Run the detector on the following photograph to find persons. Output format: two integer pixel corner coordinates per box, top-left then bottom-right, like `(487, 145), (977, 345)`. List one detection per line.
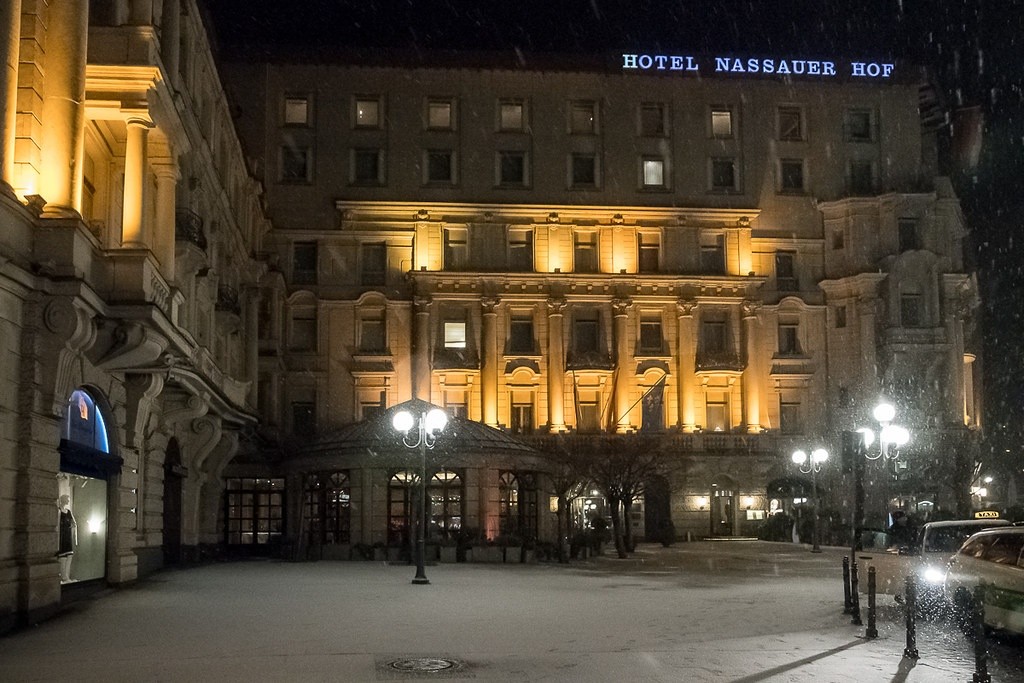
(57, 495), (78, 582)
(757, 504), (934, 551)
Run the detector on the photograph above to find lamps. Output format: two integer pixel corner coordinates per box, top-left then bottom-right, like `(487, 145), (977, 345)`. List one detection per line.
(89, 219), (106, 237)
(24, 195), (47, 217)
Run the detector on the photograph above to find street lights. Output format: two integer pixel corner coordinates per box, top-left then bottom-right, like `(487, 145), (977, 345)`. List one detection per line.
(792, 448), (828, 554)
(392, 408), (450, 587)
(854, 403), (909, 534)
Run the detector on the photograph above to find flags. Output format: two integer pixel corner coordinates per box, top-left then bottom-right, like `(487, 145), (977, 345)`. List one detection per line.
(642, 377), (665, 430)
(575, 387), (583, 421)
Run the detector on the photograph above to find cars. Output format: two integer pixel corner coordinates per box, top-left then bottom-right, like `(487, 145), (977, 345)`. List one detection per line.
(853, 511), (1024, 636)
